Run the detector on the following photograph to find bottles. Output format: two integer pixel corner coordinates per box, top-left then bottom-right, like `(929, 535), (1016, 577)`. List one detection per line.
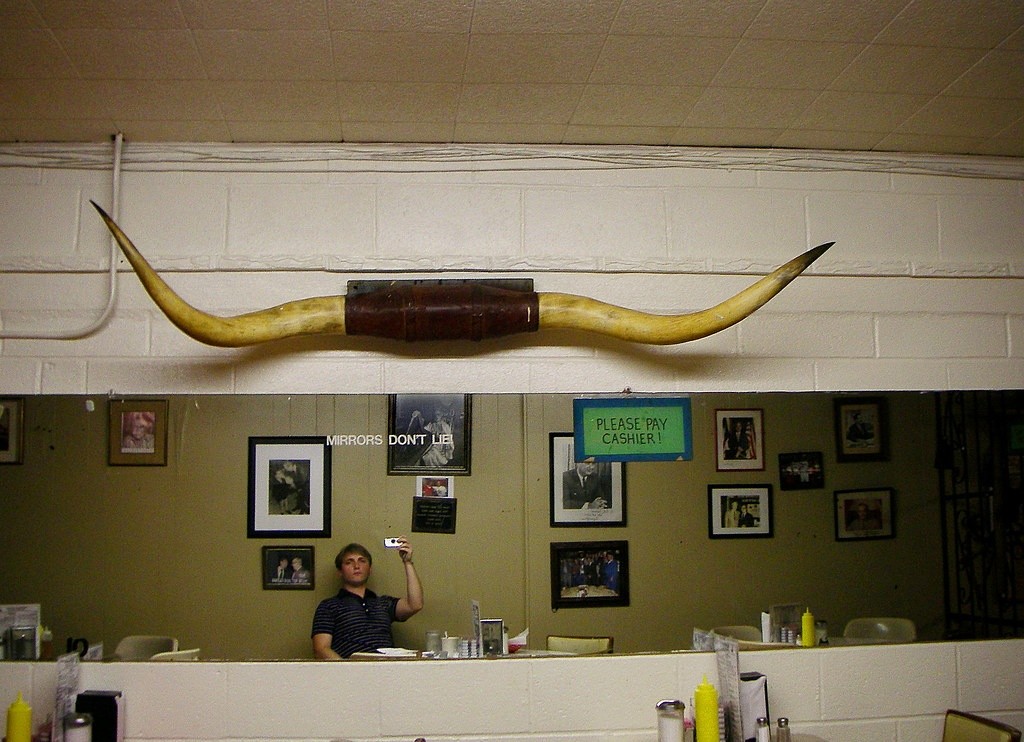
(802, 606), (814, 648)
(654, 698), (686, 742)
(4, 691), (33, 742)
(814, 619), (828, 647)
(63, 713), (92, 742)
(754, 717), (772, 742)
(40, 626), (54, 658)
(776, 718), (792, 742)
(694, 673), (720, 742)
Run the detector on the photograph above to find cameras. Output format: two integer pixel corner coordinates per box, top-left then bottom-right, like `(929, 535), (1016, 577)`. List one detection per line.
(384, 537), (404, 548)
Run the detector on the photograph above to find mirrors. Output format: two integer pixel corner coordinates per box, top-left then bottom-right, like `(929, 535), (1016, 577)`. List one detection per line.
(0, 387), (1024, 663)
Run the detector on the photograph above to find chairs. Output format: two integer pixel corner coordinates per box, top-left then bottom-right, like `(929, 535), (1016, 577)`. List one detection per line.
(111, 634), (202, 663)
(710, 616), (918, 647)
(547, 633), (614, 654)
(942, 709), (1022, 742)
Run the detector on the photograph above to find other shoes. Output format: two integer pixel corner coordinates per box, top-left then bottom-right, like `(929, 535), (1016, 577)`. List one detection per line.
(292, 507), (298, 511)
(299, 511), (304, 514)
(281, 513), (284, 515)
(286, 509), (291, 514)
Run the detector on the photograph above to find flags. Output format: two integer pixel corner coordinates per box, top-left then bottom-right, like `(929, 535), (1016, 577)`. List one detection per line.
(744, 417), (756, 458)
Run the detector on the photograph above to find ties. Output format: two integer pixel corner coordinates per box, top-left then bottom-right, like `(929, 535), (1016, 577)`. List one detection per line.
(280, 569), (283, 580)
(582, 478), (586, 488)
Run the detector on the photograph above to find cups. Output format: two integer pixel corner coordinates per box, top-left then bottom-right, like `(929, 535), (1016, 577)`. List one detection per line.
(441, 636), (458, 654)
(425, 630), (440, 653)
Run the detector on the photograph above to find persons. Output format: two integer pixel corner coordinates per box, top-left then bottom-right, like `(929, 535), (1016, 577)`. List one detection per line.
(121, 414), (154, 449)
(847, 503), (881, 531)
(560, 549), (618, 594)
(562, 460), (609, 509)
(421, 408), (455, 466)
(847, 412), (873, 443)
(268, 558), (311, 583)
(724, 501), (755, 528)
(729, 420), (750, 460)
(272, 462), (310, 515)
(311, 534), (424, 660)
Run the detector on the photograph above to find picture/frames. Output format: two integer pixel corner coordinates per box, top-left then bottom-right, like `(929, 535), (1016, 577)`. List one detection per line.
(416, 475), (454, 498)
(386, 393), (472, 478)
(0, 394), (25, 465)
(108, 399), (168, 467)
(247, 435), (332, 538)
(831, 396), (892, 463)
(550, 540), (630, 609)
(832, 488), (896, 542)
(549, 431), (628, 528)
(261, 545), (315, 591)
(707, 483), (774, 539)
(779, 451), (824, 492)
(715, 407), (765, 472)
